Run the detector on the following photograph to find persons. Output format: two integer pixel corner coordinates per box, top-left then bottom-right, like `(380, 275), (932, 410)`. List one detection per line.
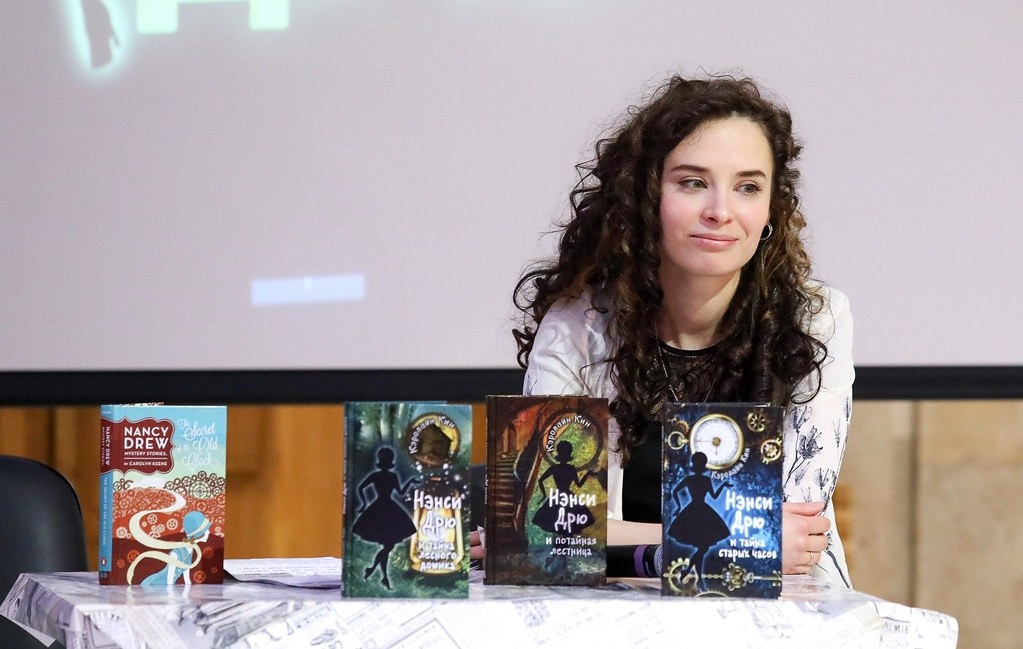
(513, 71), (855, 593)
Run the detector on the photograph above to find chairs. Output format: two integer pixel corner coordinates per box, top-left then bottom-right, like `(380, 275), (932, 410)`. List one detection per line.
(0, 454), (88, 649)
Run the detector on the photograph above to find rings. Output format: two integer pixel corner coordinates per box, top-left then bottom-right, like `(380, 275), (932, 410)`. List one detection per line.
(806, 551), (814, 565)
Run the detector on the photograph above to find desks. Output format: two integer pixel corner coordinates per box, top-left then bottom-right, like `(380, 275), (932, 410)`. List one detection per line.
(1, 565), (960, 649)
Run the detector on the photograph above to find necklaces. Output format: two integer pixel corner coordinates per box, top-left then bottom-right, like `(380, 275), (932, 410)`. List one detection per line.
(653, 315), (736, 404)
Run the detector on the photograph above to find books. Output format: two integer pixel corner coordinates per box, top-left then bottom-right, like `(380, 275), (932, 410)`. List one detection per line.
(98, 406), (229, 589)
(661, 400), (785, 599)
(484, 394), (613, 587)
(338, 399), (474, 600)
